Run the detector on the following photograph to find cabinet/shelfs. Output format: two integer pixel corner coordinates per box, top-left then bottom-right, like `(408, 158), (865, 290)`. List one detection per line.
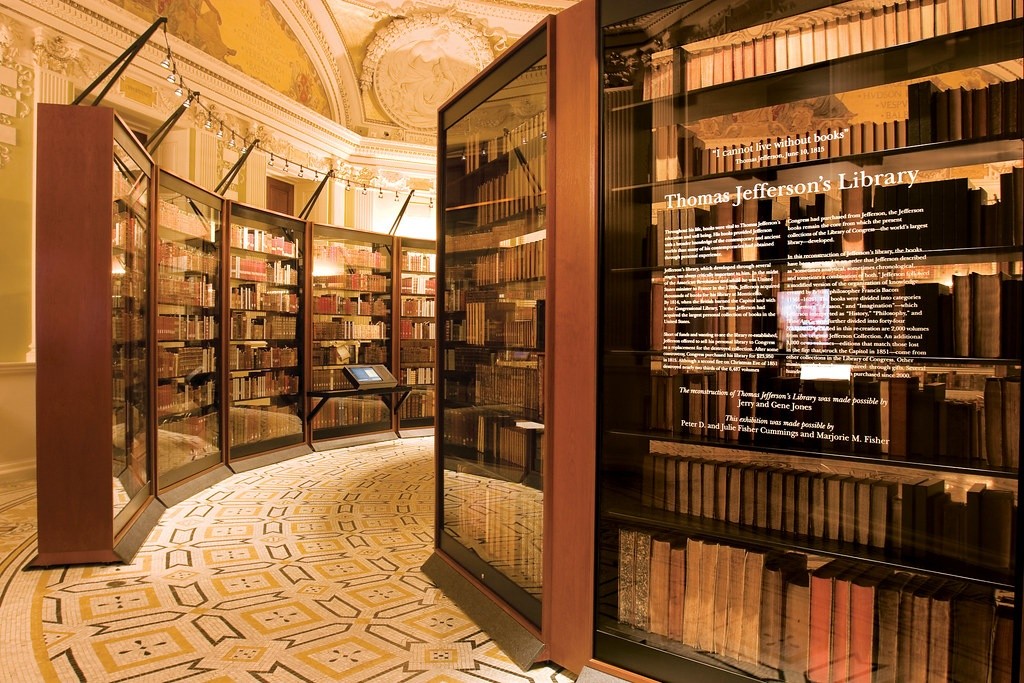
(417, 0), (1024, 683)
(308, 220), (400, 452)
(22, 102), (166, 571)
(226, 199), (313, 474)
(155, 164), (235, 507)
(394, 235), (437, 439)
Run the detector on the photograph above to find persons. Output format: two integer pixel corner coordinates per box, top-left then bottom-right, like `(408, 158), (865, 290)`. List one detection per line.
(783, 290), (826, 352)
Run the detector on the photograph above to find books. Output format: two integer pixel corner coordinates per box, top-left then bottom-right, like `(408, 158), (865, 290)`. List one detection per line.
(113, 0), (1024, 683)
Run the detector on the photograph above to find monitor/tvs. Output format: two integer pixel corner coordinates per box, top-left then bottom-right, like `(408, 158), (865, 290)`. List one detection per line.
(349, 367), (383, 382)
